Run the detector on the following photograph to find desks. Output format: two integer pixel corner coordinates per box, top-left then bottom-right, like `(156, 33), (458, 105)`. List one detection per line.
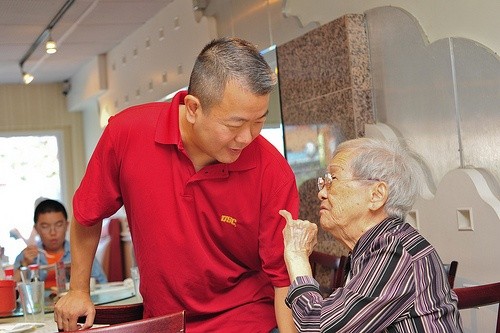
(0, 277), (147, 333)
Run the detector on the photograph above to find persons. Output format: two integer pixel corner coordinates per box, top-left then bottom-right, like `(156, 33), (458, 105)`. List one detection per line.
(54, 37), (300, 333)
(279, 137), (463, 333)
(12, 199), (107, 291)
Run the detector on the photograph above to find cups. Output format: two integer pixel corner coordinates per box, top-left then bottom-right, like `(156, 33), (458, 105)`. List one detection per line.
(17, 280), (45, 325)
(130, 266), (143, 303)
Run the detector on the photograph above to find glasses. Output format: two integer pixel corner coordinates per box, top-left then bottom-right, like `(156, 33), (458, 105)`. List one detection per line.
(318, 173), (379, 191)
(34, 222), (66, 234)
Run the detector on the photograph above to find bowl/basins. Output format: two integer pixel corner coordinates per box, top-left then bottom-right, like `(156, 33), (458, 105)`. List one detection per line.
(0, 279), (17, 317)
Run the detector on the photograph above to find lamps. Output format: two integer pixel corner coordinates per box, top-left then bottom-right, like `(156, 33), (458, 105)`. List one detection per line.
(20, 65), (34, 85)
(46, 28), (57, 54)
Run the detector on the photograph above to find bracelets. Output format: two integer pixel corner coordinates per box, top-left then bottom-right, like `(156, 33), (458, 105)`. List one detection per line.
(16, 260), (23, 271)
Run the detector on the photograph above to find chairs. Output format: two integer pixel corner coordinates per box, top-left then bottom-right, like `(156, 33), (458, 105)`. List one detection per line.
(95, 219), (125, 283)
(71, 309), (187, 333)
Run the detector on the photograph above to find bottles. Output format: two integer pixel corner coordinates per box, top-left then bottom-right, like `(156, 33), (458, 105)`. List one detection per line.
(28, 264), (43, 282)
